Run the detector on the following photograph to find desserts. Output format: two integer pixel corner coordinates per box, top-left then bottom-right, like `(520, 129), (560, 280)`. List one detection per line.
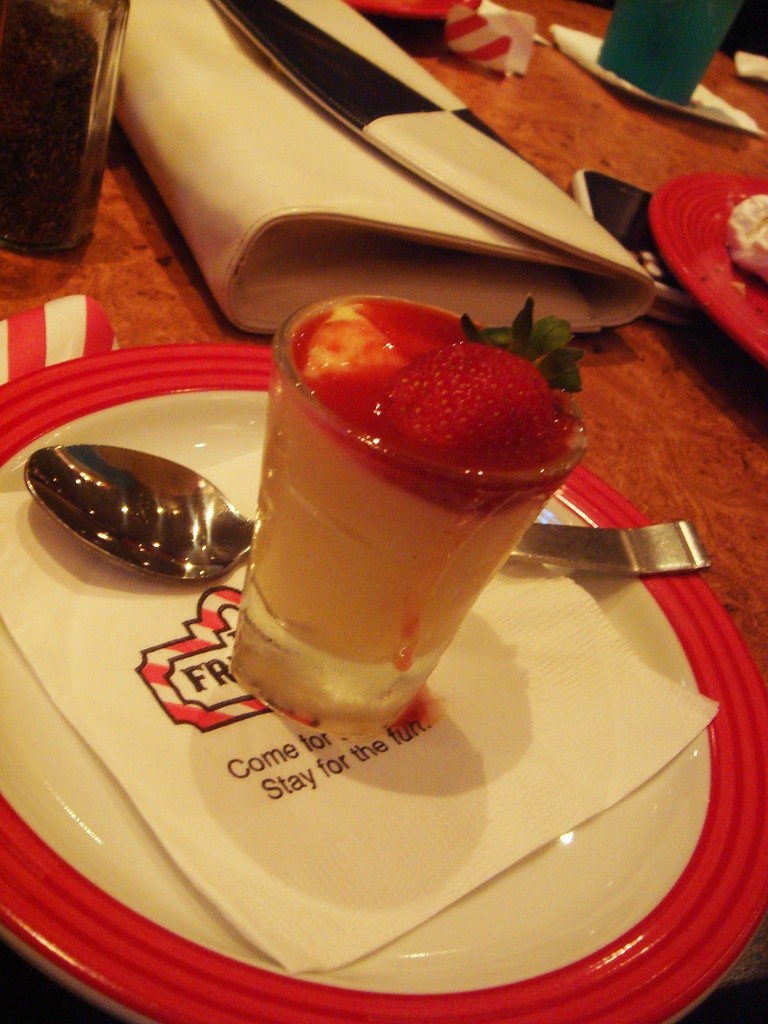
(725, 193), (768, 286)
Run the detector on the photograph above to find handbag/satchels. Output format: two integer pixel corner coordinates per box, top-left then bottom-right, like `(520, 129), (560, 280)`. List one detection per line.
(112, 1), (656, 334)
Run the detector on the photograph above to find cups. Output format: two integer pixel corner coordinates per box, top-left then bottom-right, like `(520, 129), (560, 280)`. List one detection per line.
(230, 296), (588, 737)
(0, 0), (132, 253)
(597, 0), (743, 106)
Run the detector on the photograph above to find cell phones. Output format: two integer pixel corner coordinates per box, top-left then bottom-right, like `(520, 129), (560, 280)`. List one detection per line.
(568, 167), (706, 329)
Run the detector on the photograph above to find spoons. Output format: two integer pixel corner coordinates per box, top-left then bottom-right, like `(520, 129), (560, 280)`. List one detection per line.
(24, 443), (712, 584)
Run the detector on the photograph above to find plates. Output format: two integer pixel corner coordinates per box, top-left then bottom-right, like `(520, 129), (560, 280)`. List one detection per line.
(648, 172), (768, 369)
(0, 343), (768, 1024)
(342, 0), (482, 33)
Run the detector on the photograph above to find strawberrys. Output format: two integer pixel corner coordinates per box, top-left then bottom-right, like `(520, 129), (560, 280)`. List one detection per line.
(387, 304), (586, 461)
